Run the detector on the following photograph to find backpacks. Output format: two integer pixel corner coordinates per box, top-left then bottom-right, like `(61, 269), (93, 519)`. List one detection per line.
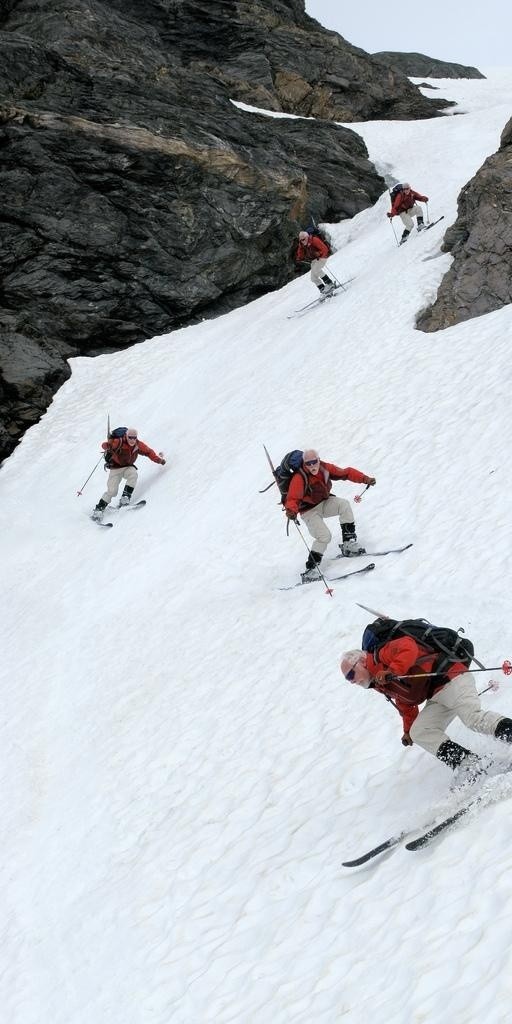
(390, 182), (413, 215)
(361, 616), (474, 701)
(275, 449), (329, 510)
(104, 426), (138, 470)
(306, 226), (331, 259)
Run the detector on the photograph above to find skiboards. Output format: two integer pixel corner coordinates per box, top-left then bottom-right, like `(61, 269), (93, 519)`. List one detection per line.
(390, 216), (442, 251)
(286, 276), (356, 319)
(340, 767), (512, 867)
(275, 545), (412, 591)
(89, 500), (146, 528)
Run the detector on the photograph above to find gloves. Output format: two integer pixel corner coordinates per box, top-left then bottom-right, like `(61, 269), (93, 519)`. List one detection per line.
(366, 478), (376, 486)
(401, 733), (413, 746)
(286, 509), (297, 520)
(375, 670), (393, 686)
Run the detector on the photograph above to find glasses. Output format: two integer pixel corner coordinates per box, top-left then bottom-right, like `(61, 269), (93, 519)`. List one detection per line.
(344, 657), (360, 681)
(127, 435), (139, 440)
(302, 459), (319, 466)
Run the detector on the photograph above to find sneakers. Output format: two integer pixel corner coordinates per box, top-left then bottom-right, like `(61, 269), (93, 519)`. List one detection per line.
(302, 568), (330, 582)
(342, 538), (367, 553)
(450, 751), (483, 792)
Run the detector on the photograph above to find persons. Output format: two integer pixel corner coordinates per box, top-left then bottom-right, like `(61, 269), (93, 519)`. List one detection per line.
(339, 617), (511, 787)
(89, 423), (166, 522)
(287, 448), (375, 578)
(385, 183), (428, 243)
(296, 230), (334, 302)
